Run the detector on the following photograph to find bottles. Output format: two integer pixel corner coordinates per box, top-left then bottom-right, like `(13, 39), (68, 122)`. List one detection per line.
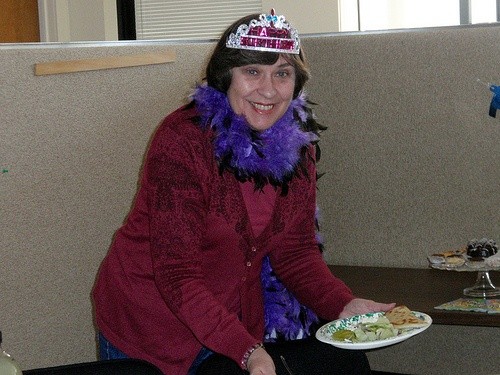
(0, 331), (23, 375)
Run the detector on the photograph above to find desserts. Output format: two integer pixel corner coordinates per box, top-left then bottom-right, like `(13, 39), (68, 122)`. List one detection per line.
(427, 238), (500, 268)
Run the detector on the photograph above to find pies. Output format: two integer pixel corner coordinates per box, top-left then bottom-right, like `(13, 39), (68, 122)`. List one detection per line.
(381, 304), (427, 325)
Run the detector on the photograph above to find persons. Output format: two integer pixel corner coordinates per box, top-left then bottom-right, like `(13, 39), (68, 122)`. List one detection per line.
(90, 8), (396, 375)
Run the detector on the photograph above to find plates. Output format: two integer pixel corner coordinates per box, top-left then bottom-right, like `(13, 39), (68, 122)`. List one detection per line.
(316, 310), (432, 348)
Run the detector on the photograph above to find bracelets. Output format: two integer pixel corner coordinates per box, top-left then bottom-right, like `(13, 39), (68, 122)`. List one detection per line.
(241, 342), (265, 371)
